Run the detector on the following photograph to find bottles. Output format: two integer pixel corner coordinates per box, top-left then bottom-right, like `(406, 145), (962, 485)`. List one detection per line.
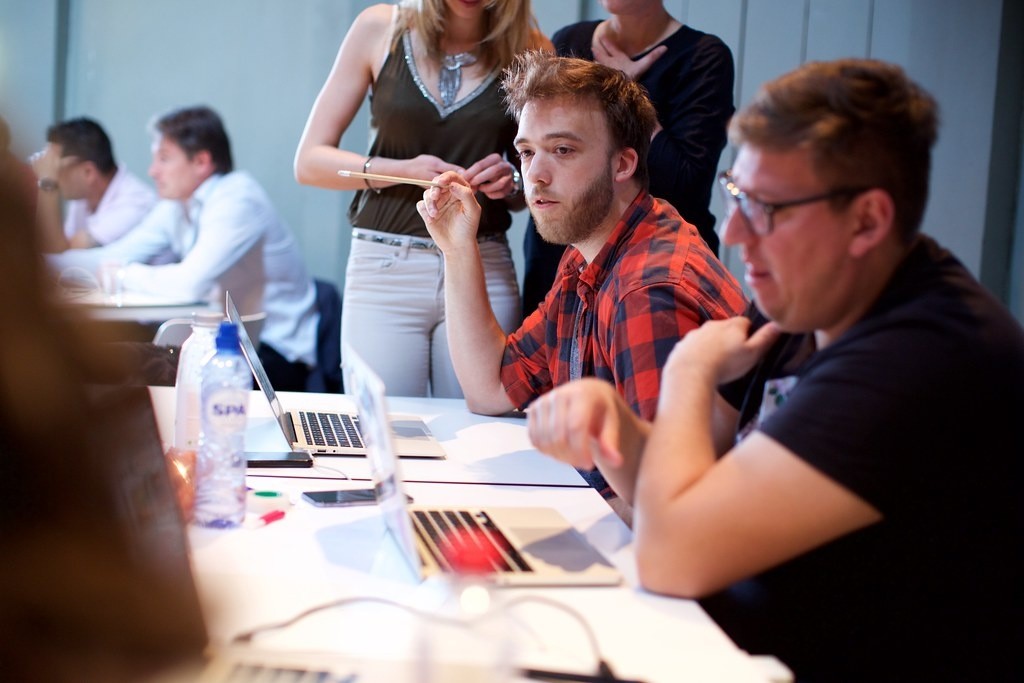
(175, 313), (225, 452)
(192, 322), (252, 527)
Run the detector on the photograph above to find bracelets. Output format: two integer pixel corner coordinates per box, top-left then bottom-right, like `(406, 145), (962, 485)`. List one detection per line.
(512, 164), (523, 191)
(37, 178), (59, 192)
(363, 156), (381, 195)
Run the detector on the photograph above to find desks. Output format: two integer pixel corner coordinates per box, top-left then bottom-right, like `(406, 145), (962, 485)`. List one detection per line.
(149, 389), (782, 682)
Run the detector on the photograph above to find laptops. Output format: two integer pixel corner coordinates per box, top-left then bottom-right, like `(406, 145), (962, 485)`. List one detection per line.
(345, 346), (625, 585)
(223, 288), (447, 463)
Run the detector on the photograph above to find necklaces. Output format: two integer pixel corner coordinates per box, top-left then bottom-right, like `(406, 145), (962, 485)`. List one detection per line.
(439, 51), (477, 106)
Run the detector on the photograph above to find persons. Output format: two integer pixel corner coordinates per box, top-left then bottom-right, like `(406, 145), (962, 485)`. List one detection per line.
(527, 54), (1024, 683)
(521, 0), (737, 328)
(417, 46), (751, 532)
(0, 147), (214, 682)
(28, 119), (183, 266)
(292, 0), (558, 400)
(44, 107), (319, 393)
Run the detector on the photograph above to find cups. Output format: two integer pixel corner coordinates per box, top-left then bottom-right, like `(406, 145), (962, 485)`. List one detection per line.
(100, 265), (126, 295)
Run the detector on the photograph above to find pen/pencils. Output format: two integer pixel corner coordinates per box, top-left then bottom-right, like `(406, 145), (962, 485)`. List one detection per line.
(252, 498), (296, 531)
(250, 486), (288, 498)
(337, 170), (450, 187)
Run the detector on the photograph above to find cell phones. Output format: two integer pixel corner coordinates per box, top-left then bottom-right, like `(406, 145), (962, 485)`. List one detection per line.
(300, 487), (411, 508)
(226, 452), (313, 469)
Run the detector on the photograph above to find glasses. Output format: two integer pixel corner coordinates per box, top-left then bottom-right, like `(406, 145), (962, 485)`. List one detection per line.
(717, 169), (868, 236)
